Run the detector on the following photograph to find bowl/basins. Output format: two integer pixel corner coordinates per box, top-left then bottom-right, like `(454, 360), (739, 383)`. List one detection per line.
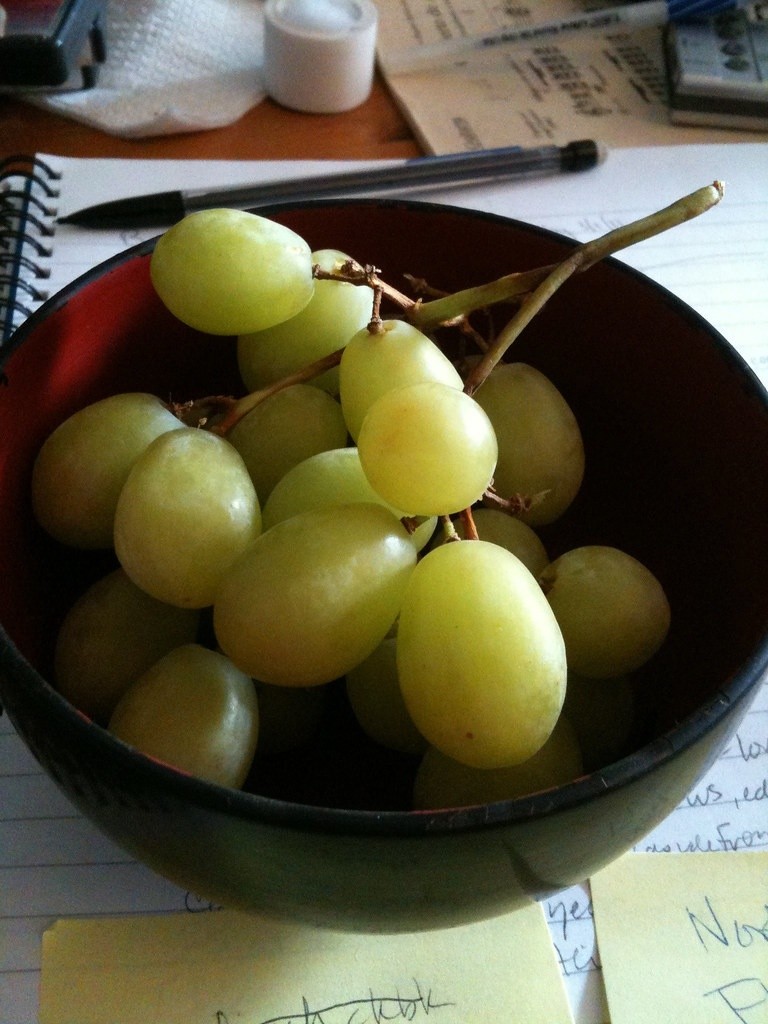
(0, 197), (766, 935)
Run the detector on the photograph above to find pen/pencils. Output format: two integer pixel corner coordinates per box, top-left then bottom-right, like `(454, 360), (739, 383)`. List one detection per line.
(390, 2), (668, 78)
(53, 137), (597, 233)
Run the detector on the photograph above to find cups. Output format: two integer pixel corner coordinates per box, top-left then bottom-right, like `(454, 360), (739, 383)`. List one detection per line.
(262, 0), (379, 115)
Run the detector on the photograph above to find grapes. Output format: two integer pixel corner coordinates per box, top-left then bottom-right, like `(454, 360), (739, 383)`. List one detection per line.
(35, 207), (671, 816)
